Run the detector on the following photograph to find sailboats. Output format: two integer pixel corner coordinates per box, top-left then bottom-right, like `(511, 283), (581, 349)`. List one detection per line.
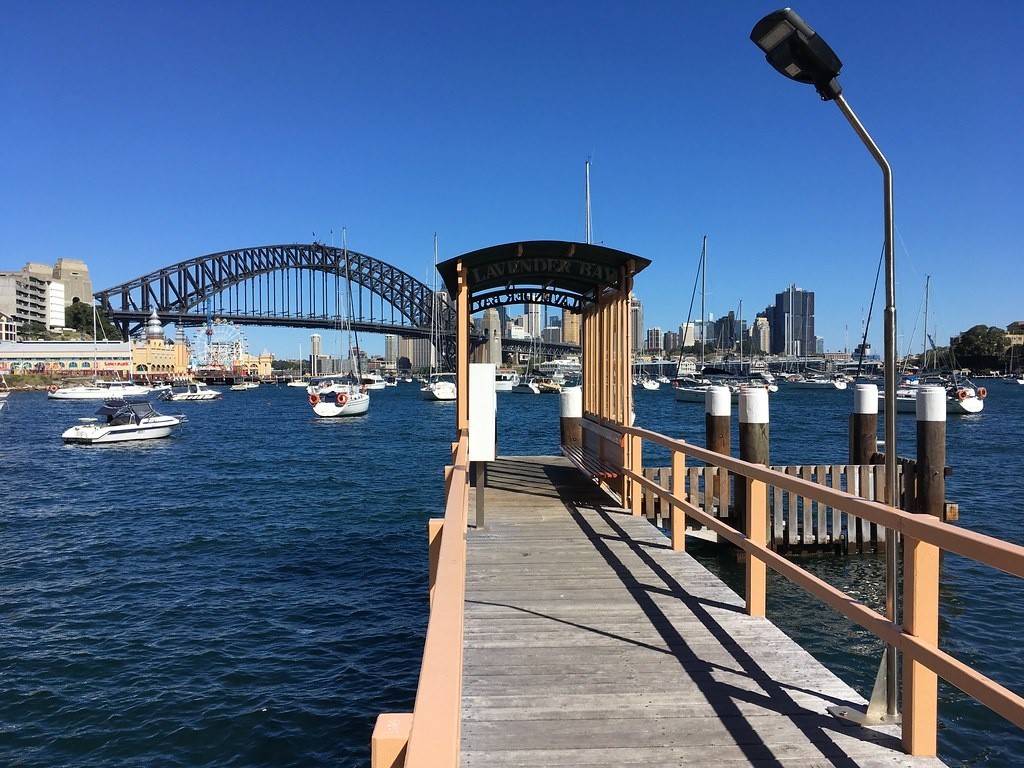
(308, 227), (370, 417)
(512, 158), (964, 401)
(1000, 330), (1024, 384)
(243, 354), (259, 389)
(418, 233), (457, 402)
(877, 275), (987, 415)
(47, 295), (172, 399)
(317, 294), (359, 394)
(287, 340), (310, 387)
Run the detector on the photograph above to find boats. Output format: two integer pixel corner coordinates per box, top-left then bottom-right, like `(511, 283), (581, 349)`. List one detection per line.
(320, 373), (386, 391)
(230, 385), (246, 391)
(495, 367), (520, 391)
(851, 317), (881, 361)
(385, 375), (424, 387)
(156, 381), (222, 401)
(61, 395), (189, 444)
(539, 360), (582, 387)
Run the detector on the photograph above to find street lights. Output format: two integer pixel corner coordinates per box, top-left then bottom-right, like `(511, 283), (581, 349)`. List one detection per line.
(750, 5), (901, 715)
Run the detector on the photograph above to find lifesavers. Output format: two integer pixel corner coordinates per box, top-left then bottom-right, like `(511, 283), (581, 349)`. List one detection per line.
(979, 388), (987, 398)
(309, 394), (319, 404)
(959, 390), (967, 400)
(51, 386), (58, 392)
(337, 394), (348, 404)
